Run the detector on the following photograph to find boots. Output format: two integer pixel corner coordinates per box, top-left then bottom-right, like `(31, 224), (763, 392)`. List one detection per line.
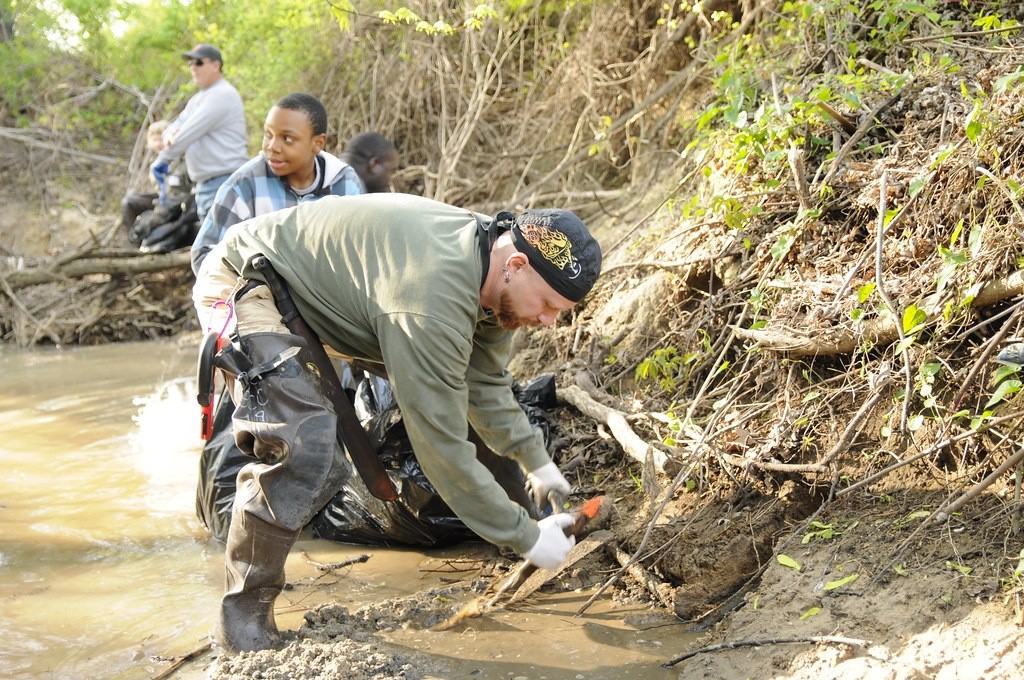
(207, 504), (302, 655)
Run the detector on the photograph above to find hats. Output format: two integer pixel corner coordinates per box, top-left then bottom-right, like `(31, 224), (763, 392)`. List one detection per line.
(489, 208), (601, 304)
(181, 44), (223, 63)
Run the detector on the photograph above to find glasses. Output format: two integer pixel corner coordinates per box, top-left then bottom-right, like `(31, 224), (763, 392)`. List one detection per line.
(187, 58), (212, 66)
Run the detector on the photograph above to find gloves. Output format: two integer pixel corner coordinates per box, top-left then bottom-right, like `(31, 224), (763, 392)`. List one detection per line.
(149, 158), (169, 186)
(524, 463), (571, 517)
(520, 512), (575, 571)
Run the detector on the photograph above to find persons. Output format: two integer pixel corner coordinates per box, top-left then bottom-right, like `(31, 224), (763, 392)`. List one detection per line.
(192, 93), (363, 277)
(341, 132), (399, 192)
(120, 121), (201, 253)
(148, 43), (250, 226)
(191, 192), (603, 657)
